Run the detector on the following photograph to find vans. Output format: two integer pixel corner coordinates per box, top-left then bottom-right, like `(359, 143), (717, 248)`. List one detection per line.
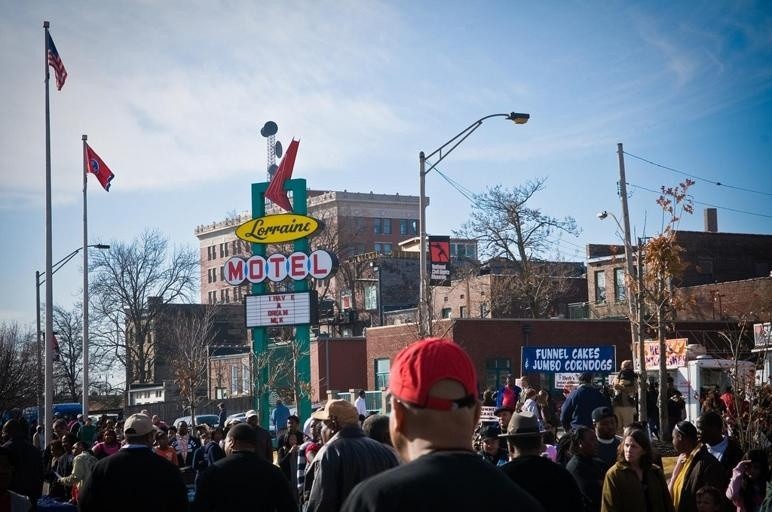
(85, 413), (118, 427)
(173, 414), (223, 430)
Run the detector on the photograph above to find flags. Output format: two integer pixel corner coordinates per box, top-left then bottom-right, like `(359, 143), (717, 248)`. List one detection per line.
(45, 30), (68, 89)
(84, 141), (115, 192)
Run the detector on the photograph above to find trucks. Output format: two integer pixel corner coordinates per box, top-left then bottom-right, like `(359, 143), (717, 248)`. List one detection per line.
(614, 360), (757, 437)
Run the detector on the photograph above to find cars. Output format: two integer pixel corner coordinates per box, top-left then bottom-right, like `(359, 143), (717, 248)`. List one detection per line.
(221, 403), (320, 432)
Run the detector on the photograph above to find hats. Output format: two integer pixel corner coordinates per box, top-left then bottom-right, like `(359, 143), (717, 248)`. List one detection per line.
(591, 407), (616, 423)
(217, 403), (224, 408)
(382, 337), (479, 411)
(311, 398), (360, 424)
(494, 406), (513, 416)
(479, 425), (501, 442)
(244, 410), (258, 419)
(497, 412), (552, 437)
(123, 413), (157, 438)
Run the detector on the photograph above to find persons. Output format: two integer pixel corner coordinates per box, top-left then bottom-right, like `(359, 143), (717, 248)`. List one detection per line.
(473, 360), (772, 512)
(341, 338), (548, 509)
(1, 388), (400, 511)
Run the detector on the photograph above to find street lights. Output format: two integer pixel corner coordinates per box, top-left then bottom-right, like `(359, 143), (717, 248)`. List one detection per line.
(34, 241), (110, 439)
(417, 112), (530, 342)
(596, 209), (627, 235)
